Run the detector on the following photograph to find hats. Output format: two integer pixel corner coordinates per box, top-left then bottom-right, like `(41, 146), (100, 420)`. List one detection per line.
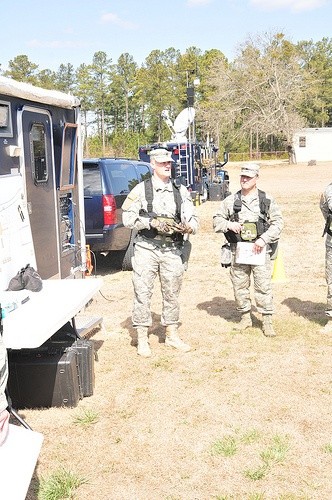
(239, 164), (259, 178)
(150, 149), (175, 163)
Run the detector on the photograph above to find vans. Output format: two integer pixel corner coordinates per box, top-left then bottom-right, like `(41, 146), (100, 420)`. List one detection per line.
(83, 157), (154, 270)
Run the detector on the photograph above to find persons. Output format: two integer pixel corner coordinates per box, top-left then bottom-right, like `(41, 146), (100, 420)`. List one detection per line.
(212, 162), (285, 337)
(320, 182), (332, 317)
(121, 148), (199, 358)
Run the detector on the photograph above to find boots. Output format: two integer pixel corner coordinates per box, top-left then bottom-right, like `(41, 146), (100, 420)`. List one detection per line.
(164, 325), (191, 352)
(261, 313), (277, 336)
(137, 329), (151, 359)
(232, 313), (253, 331)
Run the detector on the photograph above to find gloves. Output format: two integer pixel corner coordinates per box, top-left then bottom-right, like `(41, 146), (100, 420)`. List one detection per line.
(151, 220), (172, 233)
(178, 222), (192, 234)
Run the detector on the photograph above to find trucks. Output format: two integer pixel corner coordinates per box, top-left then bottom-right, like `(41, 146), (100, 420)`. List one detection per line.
(0, 75), (98, 353)
(138, 141), (229, 205)
